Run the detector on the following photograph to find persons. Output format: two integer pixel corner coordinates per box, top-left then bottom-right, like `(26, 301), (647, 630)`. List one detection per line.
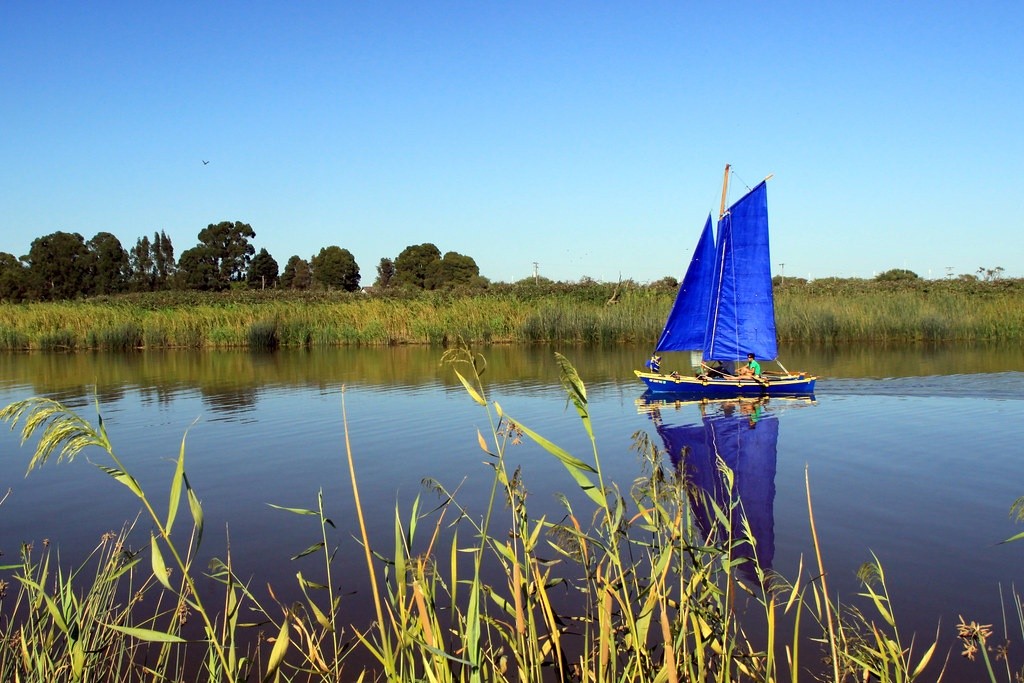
(723, 353), (760, 380)
(696, 361), (735, 380)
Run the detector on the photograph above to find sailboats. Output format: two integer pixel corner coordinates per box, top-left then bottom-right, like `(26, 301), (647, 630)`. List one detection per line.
(634, 163), (818, 393)
(631, 382), (817, 602)
